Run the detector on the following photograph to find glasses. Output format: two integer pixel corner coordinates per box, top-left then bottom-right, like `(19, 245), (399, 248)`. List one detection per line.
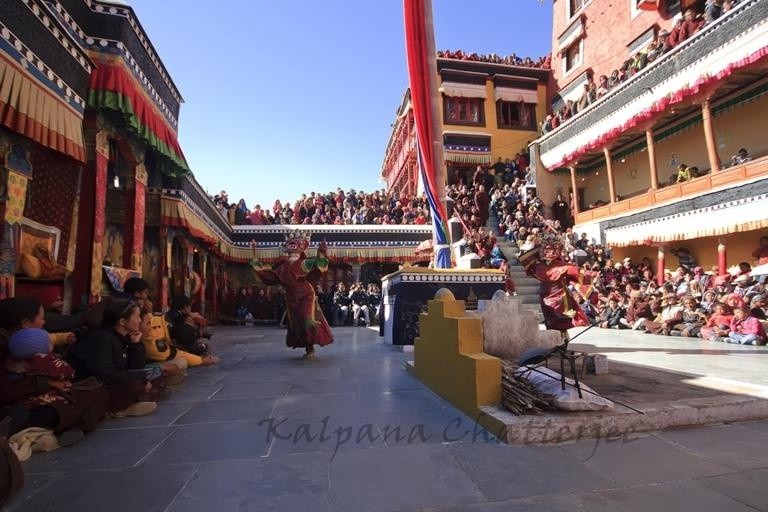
(121, 300), (135, 315)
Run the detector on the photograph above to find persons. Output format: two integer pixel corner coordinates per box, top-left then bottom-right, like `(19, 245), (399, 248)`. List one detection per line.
(437, 0), (767, 137)
(446, 137), (768, 345)
(0, 230), (228, 506)
(250, 227), (334, 361)
(210, 186), (430, 327)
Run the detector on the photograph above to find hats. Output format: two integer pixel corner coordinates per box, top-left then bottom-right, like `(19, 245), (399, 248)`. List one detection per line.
(733, 274), (749, 282)
(8, 328), (49, 359)
(630, 291), (642, 298)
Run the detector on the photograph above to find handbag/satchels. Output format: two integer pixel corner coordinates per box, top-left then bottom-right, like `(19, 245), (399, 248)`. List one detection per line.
(7, 427), (57, 462)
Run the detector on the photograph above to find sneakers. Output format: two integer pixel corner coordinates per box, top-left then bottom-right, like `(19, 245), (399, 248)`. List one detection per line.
(56, 430), (83, 446)
(751, 340), (760, 346)
(669, 330), (680, 336)
(111, 401), (157, 418)
(723, 338), (733, 343)
